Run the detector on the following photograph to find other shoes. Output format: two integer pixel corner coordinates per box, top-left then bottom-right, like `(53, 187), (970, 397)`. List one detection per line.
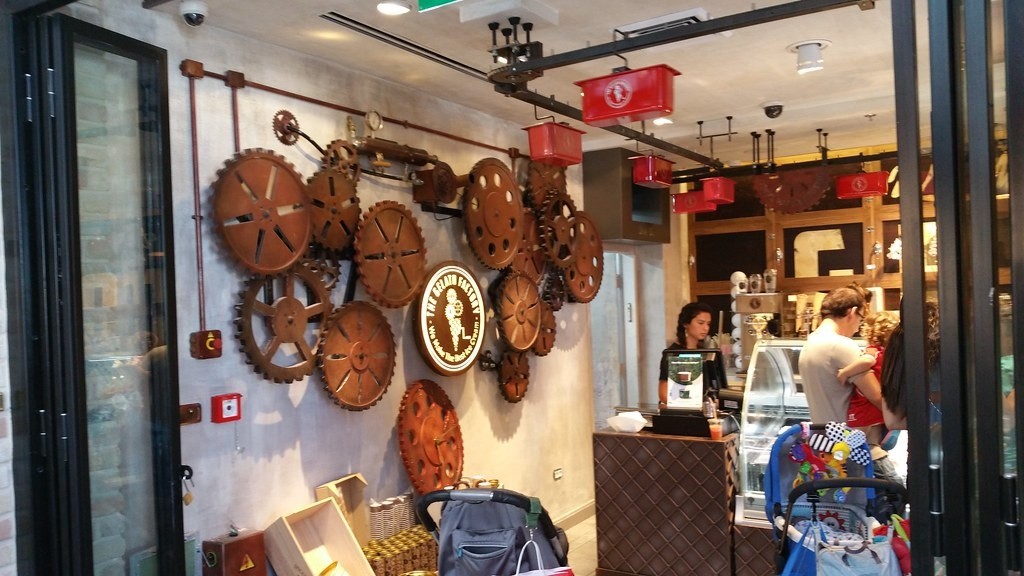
(871, 447), (886, 462)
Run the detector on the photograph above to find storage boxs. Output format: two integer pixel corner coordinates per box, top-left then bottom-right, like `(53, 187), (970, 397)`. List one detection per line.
(318, 472), (368, 548)
(260, 499), (375, 576)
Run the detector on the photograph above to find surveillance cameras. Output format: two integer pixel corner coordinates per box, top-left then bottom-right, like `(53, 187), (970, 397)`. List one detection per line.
(762, 101), (786, 118)
(179, 0), (208, 26)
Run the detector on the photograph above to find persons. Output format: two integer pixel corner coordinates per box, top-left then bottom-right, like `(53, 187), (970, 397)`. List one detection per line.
(798, 287), (903, 506)
(836, 310), (900, 438)
(658, 303), (728, 405)
(881, 293), (1020, 576)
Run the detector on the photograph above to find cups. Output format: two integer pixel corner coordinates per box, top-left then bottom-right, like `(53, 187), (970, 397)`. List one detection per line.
(707, 419), (724, 440)
(703, 401), (717, 418)
(763, 268), (776, 293)
(749, 273), (762, 293)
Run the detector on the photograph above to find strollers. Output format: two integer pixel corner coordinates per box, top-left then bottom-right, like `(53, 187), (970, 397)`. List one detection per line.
(763, 421), (911, 576)
(416, 486), (575, 576)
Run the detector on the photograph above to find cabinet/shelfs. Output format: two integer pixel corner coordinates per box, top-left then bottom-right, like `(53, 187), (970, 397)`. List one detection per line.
(688, 148), (1011, 336)
(580, 146), (671, 246)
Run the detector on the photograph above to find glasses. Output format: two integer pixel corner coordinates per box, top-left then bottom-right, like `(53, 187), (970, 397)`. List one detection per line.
(857, 311), (865, 325)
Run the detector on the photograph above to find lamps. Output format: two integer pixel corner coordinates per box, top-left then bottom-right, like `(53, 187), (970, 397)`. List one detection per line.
(797, 44), (824, 74)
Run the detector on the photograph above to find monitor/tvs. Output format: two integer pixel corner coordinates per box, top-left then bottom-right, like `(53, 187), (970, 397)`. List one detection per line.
(662, 348), (727, 400)
